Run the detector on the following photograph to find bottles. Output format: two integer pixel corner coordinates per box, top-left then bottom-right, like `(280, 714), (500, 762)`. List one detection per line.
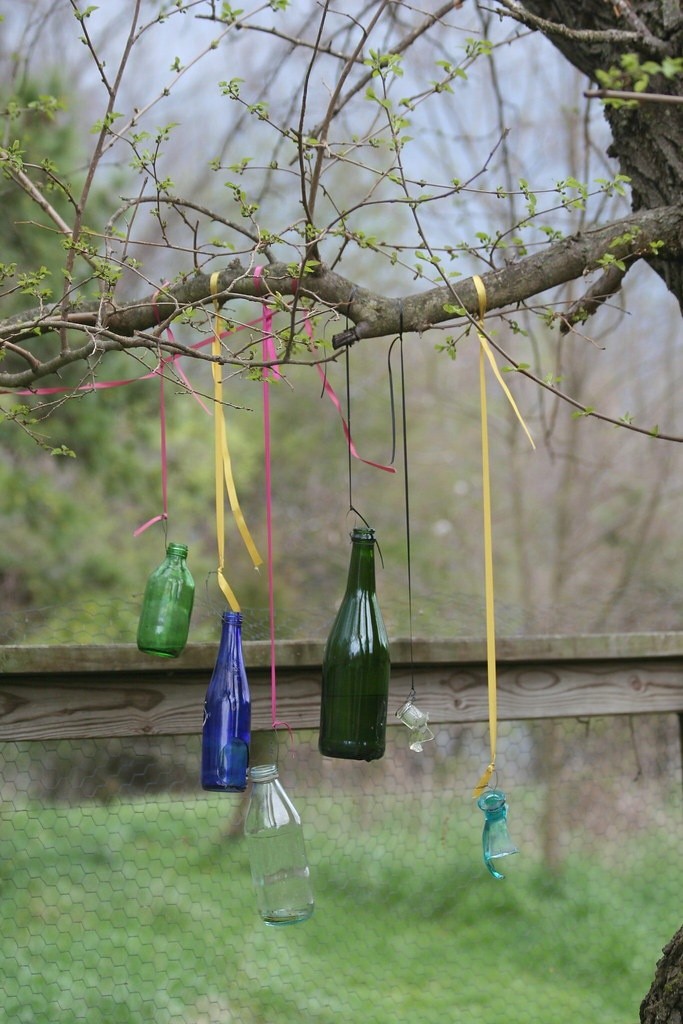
(137, 543), (195, 658)
(318, 528), (390, 762)
(201, 611), (251, 792)
(245, 764), (315, 927)
